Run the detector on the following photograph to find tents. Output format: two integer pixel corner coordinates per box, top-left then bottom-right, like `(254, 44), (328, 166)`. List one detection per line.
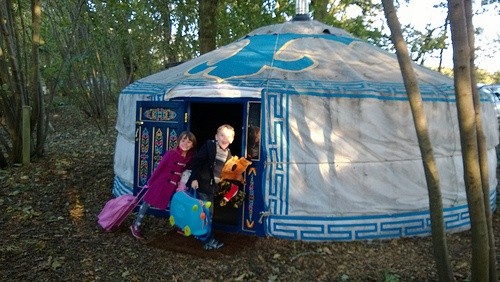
(113, 13), (499, 241)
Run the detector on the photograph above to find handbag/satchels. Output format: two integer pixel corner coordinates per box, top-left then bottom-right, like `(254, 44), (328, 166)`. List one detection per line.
(169, 188), (212, 241)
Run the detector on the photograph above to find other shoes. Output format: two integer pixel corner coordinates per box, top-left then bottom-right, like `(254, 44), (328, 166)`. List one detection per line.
(177, 228), (184, 235)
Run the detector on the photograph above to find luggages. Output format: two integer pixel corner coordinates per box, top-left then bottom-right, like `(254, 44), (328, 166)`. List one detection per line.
(97, 185), (150, 231)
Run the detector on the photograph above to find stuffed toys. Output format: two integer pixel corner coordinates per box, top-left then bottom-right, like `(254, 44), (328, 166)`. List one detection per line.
(214, 155), (253, 208)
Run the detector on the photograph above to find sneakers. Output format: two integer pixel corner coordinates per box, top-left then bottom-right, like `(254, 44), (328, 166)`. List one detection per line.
(130, 225), (142, 238)
(203, 239), (224, 249)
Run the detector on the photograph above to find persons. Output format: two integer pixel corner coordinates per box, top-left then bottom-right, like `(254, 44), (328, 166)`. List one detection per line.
(129, 131), (196, 239)
(185, 125), (235, 251)
(238, 125), (260, 159)
